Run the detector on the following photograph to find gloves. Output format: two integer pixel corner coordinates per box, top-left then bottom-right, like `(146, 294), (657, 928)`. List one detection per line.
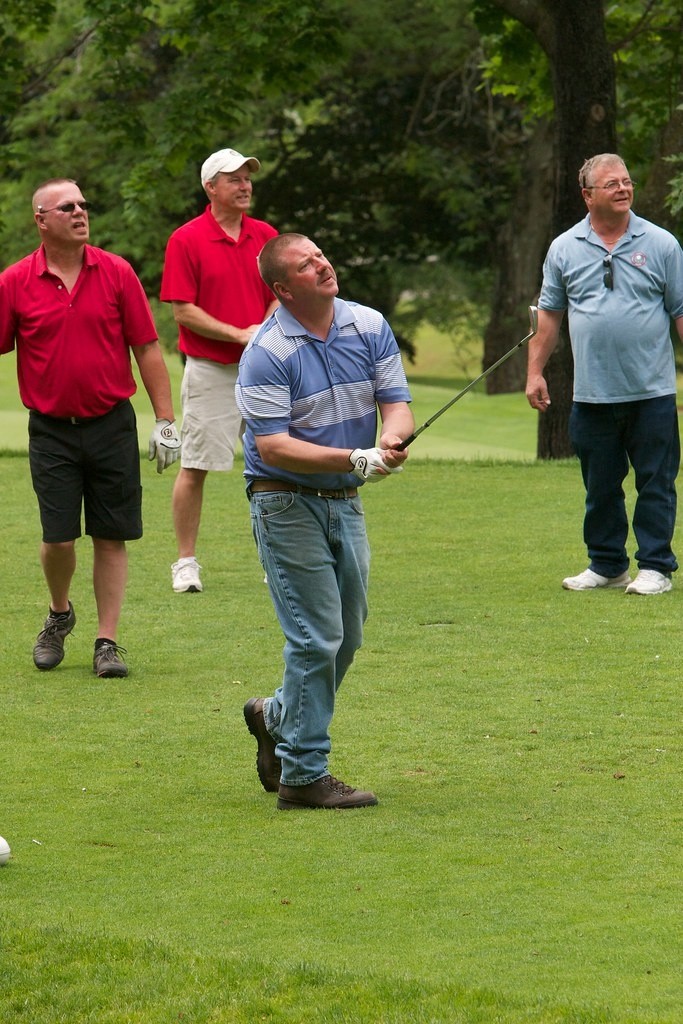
(349, 448), (403, 484)
(148, 418), (181, 474)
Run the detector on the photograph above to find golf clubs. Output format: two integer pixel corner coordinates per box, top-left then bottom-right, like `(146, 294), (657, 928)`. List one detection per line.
(391, 307), (539, 453)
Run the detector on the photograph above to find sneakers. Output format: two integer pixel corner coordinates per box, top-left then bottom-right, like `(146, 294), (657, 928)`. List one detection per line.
(170, 558), (203, 593)
(33, 599), (76, 670)
(561, 568), (632, 591)
(276, 774), (378, 810)
(243, 697), (284, 793)
(92, 643), (128, 677)
(625, 568), (672, 596)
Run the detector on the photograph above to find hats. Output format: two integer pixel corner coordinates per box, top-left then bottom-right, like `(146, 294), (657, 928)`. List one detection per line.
(201, 148), (260, 190)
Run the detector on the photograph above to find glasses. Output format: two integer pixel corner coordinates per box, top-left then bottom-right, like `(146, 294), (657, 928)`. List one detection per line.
(587, 181), (637, 191)
(602, 253), (612, 291)
(40, 201), (92, 213)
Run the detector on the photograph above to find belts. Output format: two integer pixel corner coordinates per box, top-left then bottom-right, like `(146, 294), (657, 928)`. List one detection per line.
(249, 479), (359, 501)
(36, 412), (112, 427)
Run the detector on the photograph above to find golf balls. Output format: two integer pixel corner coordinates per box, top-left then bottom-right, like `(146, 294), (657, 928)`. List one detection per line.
(0, 834), (12, 866)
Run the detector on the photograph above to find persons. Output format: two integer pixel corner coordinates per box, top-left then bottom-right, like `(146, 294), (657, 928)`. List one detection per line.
(161, 149), (280, 592)
(0, 181), (183, 678)
(234, 234), (416, 808)
(525, 153), (683, 597)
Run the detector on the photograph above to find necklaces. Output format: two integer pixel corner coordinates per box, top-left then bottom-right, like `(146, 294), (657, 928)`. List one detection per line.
(591, 224), (624, 244)
